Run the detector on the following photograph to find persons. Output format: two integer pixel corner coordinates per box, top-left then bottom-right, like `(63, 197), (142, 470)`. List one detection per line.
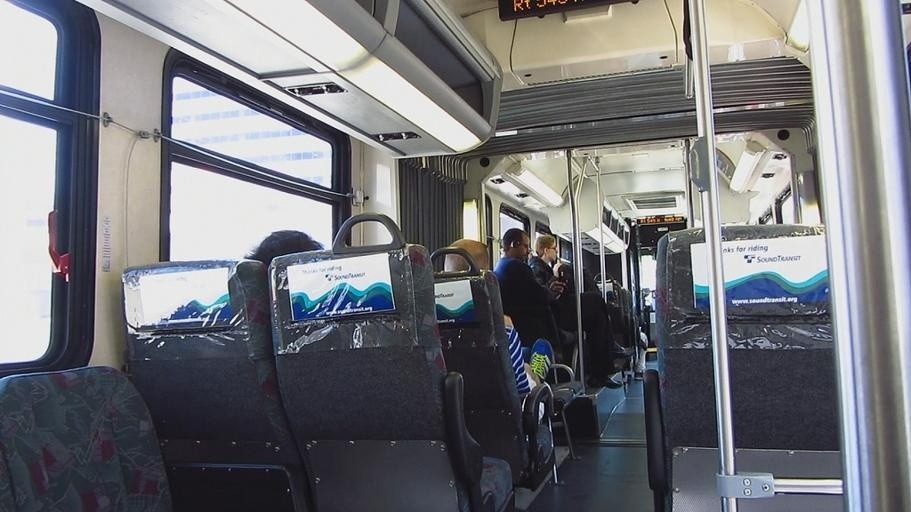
(495, 228), (649, 389)
(242, 230), (326, 268)
(443, 239), (554, 441)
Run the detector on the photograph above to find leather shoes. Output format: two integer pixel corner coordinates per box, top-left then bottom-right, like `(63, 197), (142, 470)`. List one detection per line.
(607, 340), (635, 359)
(587, 372), (623, 389)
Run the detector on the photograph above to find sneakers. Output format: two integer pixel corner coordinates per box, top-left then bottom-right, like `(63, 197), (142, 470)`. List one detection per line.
(529, 336), (554, 381)
(634, 372), (644, 380)
